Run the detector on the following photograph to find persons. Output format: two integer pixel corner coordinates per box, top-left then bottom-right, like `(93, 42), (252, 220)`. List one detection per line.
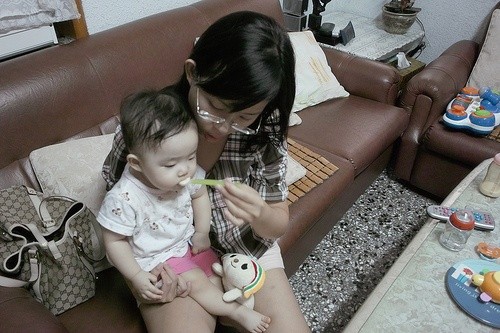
(96, 88), (272, 333)
(101, 10), (316, 333)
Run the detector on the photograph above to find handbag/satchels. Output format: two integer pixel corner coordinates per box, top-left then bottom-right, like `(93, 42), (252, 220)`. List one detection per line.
(1, 184), (107, 316)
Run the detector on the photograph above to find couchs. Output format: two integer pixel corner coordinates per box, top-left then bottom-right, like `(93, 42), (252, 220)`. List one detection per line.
(0, 0), (407, 333)
(388, 0), (500, 202)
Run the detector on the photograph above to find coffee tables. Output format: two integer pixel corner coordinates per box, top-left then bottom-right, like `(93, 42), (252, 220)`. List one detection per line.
(341, 156), (500, 333)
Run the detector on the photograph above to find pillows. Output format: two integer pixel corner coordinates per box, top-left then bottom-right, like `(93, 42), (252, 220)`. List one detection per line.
(195, 31), (349, 121)
(29, 132), (119, 264)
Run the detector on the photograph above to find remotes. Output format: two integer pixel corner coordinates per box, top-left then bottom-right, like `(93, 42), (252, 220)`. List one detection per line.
(427, 205), (496, 231)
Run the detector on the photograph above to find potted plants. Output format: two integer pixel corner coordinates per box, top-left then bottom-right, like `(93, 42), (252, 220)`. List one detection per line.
(383, 0), (421, 34)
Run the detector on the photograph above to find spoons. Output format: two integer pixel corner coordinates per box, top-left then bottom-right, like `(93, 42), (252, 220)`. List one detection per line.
(178, 176), (230, 190)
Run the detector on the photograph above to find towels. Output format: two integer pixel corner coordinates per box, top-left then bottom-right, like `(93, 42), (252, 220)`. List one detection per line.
(0, 0), (82, 34)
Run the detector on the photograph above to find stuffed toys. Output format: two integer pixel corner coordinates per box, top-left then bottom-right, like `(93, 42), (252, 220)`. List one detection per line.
(211, 253), (266, 332)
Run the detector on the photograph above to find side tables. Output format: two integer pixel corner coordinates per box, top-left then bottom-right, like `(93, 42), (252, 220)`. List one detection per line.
(312, 12), (426, 65)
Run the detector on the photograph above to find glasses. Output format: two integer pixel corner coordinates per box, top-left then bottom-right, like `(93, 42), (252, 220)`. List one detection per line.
(195, 75), (263, 136)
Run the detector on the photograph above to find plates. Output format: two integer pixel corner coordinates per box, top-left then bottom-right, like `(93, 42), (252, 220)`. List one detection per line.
(444, 260), (500, 329)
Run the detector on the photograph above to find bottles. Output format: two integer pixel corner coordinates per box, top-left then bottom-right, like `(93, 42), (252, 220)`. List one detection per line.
(480, 150), (500, 197)
